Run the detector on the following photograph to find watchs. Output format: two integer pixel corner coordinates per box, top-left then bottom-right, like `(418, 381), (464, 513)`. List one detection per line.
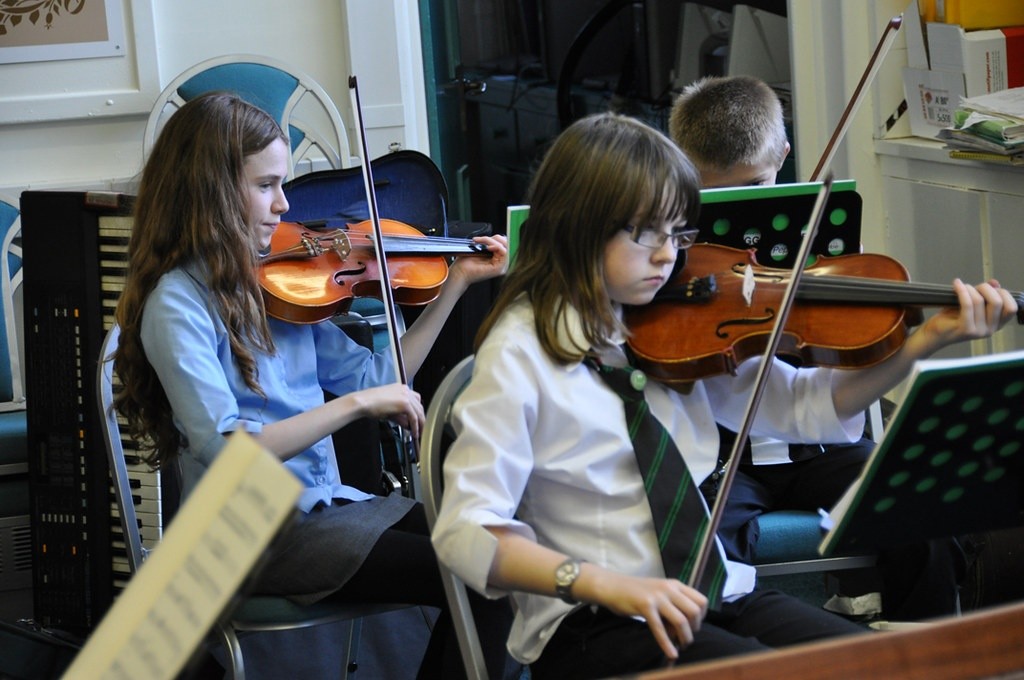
(552, 556), (586, 604)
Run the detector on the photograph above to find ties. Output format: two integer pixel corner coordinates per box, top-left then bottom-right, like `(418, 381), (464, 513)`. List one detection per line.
(581, 340), (730, 618)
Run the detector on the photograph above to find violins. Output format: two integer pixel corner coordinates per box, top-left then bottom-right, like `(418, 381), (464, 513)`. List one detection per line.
(620, 243), (1023, 384)
(256, 218), (495, 325)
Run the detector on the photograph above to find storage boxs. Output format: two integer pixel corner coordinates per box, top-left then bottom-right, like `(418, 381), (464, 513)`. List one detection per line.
(901, 0), (1024, 142)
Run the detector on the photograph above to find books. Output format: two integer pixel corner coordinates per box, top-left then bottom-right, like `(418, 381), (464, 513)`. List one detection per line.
(940, 85), (1024, 163)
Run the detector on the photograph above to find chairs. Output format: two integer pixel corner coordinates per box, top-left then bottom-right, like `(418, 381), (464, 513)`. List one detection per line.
(751, 400), (960, 620)
(141, 52), (349, 182)
(0, 192), (28, 474)
(98, 320), (435, 680)
(419, 352), (518, 680)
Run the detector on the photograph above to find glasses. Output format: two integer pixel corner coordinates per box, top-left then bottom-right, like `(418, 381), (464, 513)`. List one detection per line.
(621, 223), (699, 249)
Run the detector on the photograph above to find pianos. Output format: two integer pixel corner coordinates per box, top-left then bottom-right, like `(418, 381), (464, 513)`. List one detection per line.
(19, 190), (162, 646)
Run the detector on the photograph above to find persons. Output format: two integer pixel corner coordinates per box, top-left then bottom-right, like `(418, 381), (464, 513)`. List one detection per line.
(431, 111), (1018, 679)
(102, 91), (517, 680)
(663, 70), (958, 622)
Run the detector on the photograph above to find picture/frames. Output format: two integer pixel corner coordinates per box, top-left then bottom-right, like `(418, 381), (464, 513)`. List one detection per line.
(0, 0), (162, 124)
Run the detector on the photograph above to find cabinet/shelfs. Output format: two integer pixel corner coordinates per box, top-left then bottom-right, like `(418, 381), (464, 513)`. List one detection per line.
(476, 81), (671, 236)
(875, 136), (1023, 407)
